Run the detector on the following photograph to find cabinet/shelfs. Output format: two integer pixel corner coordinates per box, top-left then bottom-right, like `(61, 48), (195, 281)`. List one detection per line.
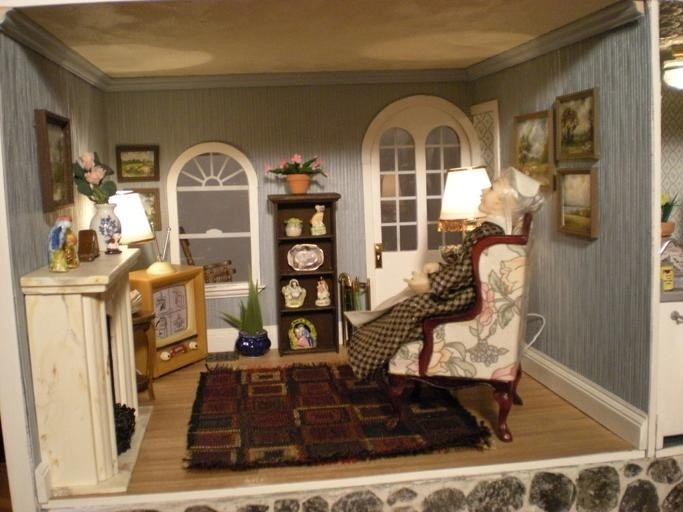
(267, 190), (342, 358)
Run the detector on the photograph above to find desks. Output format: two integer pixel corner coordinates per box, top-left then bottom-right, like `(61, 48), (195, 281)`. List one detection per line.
(127, 311), (157, 401)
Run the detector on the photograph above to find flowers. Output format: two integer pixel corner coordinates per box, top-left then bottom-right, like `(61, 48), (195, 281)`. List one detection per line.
(70, 152), (116, 204)
(264, 153), (328, 178)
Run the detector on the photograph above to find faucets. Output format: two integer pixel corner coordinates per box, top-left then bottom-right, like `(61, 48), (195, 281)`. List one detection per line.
(660, 238), (678, 256)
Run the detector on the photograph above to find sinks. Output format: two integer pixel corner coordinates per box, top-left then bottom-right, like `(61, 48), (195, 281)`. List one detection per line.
(661, 261), (682, 278)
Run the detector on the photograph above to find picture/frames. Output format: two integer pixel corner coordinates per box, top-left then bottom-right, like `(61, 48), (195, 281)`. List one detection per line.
(113, 144), (161, 182)
(130, 187), (162, 231)
(509, 107), (555, 195)
(551, 166), (597, 243)
(35, 109), (76, 211)
(552, 86), (600, 161)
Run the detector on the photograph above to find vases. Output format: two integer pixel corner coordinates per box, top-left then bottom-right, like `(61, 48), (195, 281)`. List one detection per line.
(285, 173), (310, 194)
(89, 202), (121, 252)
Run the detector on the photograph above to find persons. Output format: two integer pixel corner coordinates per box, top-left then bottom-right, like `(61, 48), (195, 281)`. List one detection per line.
(347, 168), (545, 388)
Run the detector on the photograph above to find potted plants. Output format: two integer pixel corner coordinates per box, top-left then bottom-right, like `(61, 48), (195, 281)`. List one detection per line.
(225, 278), (271, 358)
(281, 216), (304, 237)
(661, 193), (681, 242)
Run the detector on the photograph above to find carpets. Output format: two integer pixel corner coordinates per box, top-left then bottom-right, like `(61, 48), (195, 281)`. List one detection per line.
(184, 359), (490, 470)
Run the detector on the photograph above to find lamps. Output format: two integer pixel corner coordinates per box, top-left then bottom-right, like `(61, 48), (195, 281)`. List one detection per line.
(436, 166), (492, 243)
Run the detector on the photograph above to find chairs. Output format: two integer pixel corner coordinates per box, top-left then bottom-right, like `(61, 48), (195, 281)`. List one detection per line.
(386, 214), (532, 442)
(178, 226), (236, 283)
